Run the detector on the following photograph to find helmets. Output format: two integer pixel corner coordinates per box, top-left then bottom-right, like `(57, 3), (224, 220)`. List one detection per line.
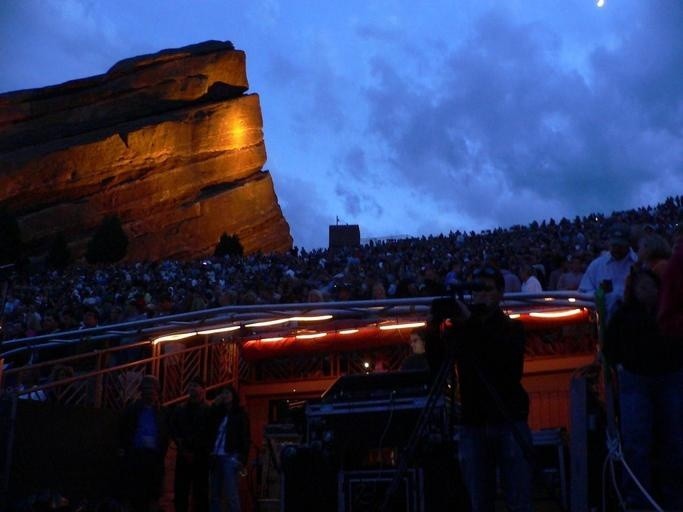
(471, 264), (506, 295)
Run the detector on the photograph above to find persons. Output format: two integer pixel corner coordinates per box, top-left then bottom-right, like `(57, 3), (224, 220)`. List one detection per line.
(2, 194), (682, 511)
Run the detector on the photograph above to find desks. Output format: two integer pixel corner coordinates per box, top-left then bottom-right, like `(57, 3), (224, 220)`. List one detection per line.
(532, 427), (569, 512)
